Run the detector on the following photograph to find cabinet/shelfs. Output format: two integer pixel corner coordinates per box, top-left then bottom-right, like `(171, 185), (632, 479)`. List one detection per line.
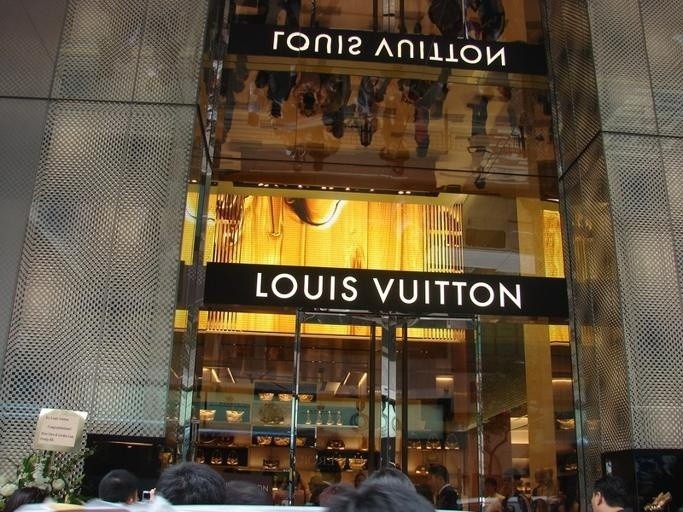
(170, 373), (471, 493)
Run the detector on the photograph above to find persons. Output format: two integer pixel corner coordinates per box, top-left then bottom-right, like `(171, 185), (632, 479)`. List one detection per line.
(319, 483), (356, 507)
(555, 473), (580, 512)
(591, 476), (634, 512)
(415, 465), (434, 504)
(309, 476), (322, 493)
(542, 467), (558, 512)
(225, 481), (274, 506)
(226, 1), (551, 189)
(99, 470), (154, 506)
(532, 468), (546, 512)
(354, 474), (366, 488)
(428, 463), (463, 511)
(330, 487), (435, 512)
(309, 460), (341, 506)
(502, 468), (534, 512)
(357, 467), (416, 493)
(484, 477), (505, 511)
(157, 464), (226, 505)
(4, 488), (44, 512)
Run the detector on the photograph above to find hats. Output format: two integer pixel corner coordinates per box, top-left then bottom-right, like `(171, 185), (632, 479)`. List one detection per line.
(502, 468), (521, 479)
